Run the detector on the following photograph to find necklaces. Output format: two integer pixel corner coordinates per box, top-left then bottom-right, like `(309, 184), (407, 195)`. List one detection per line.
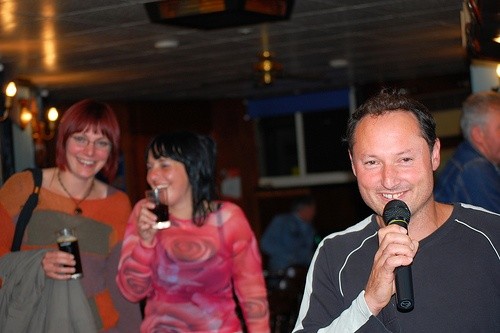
(58, 171), (95, 216)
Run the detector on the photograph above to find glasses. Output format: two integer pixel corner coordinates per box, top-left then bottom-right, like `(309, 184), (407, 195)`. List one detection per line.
(69, 135), (111, 149)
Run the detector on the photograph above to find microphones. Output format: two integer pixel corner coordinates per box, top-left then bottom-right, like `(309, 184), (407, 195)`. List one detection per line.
(382, 199), (415, 313)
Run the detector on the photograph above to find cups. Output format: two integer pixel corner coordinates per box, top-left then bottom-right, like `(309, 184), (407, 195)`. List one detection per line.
(145, 188), (171, 229)
(55, 227), (83, 279)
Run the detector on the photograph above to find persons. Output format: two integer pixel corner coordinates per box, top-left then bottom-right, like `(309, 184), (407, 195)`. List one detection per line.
(434, 90), (500, 216)
(260, 196), (317, 292)
(290, 89), (500, 333)
(0, 98), (143, 333)
(114, 130), (272, 333)
